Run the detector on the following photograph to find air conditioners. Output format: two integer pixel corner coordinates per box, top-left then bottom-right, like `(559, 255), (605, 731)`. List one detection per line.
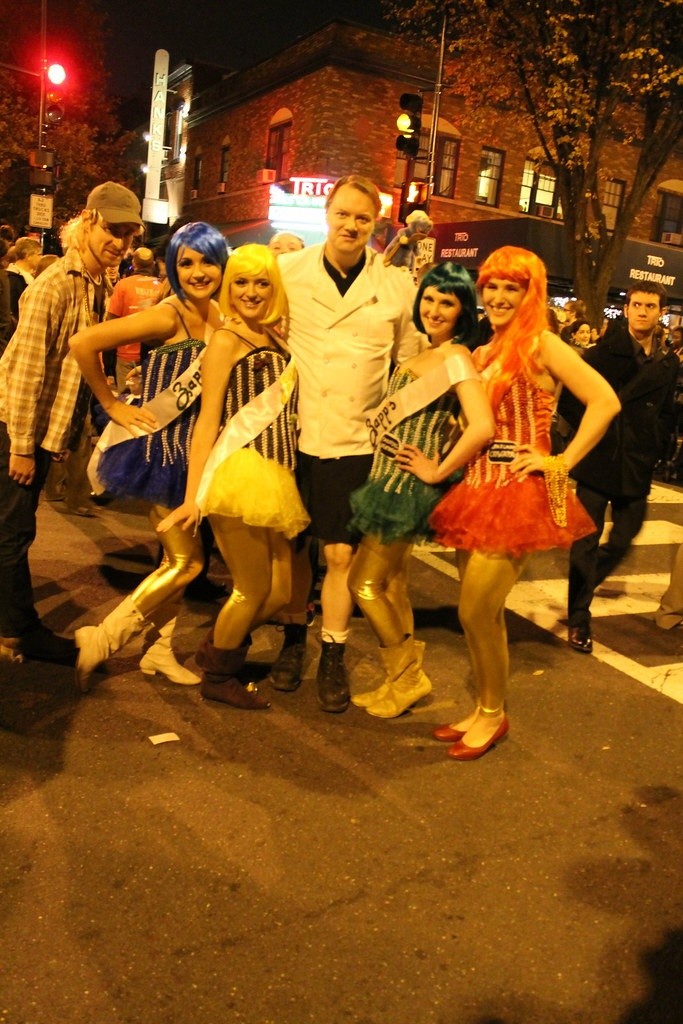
(538, 206), (554, 219)
(217, 183), (225, 193)
(256, 169), (276, 185)
(190, 189), (197, 200)
(661, 232), (682, 246)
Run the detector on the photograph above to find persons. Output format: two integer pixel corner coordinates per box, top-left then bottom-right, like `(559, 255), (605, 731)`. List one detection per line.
(0, 180), (147, 665)
(271, 175), (418, 712)
(655, 327), (683, 482)
(556, 282), (680, 653)
(269, 231), (305, 260)
(427, 244), (622, 762)
(1, 225), (168, 464)
(157, 243), (313, 711)
(547, 299), (683, 377)
(68, 220), (236, 693)
(347, 258), (496, 719)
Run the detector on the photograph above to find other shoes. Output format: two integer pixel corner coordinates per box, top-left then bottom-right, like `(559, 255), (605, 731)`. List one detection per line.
(16, 625), (80, 663)
(184, 575), (226, 603)
(433, 708), (489, 742)
(450, 708), (508, 761)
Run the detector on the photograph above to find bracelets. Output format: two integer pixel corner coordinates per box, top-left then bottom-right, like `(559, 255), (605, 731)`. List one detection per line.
(543, 453), (569, 528)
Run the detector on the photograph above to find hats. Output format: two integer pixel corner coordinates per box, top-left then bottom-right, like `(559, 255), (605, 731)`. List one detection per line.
(87, 180), (148, 232)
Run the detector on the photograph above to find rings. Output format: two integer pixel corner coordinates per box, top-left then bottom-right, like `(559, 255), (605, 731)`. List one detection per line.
(406, 458), (410, 464)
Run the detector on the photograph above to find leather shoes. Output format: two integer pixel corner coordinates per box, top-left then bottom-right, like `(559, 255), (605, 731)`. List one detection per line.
(569, 619), (592, 653)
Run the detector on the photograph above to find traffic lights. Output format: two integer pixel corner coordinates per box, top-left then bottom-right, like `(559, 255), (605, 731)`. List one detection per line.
(45, 60), (68, 125)
(30, 170), (53, 189)
(29, 150), (54, 168)
(399, 182), (426, 223)
(395, 92), (423, 155)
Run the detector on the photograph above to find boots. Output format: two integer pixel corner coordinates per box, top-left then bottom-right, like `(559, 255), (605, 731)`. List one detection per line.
(349, 638), (427, 708)
(202, 633), (272, 710)
(75, 594), (147, 695)
(140, 599), (202, 686)
(270, 620), (309, 694)
(196, 619), (253, 677)
(314, 632), (349, 714)
(366, 632), (431, 719)
